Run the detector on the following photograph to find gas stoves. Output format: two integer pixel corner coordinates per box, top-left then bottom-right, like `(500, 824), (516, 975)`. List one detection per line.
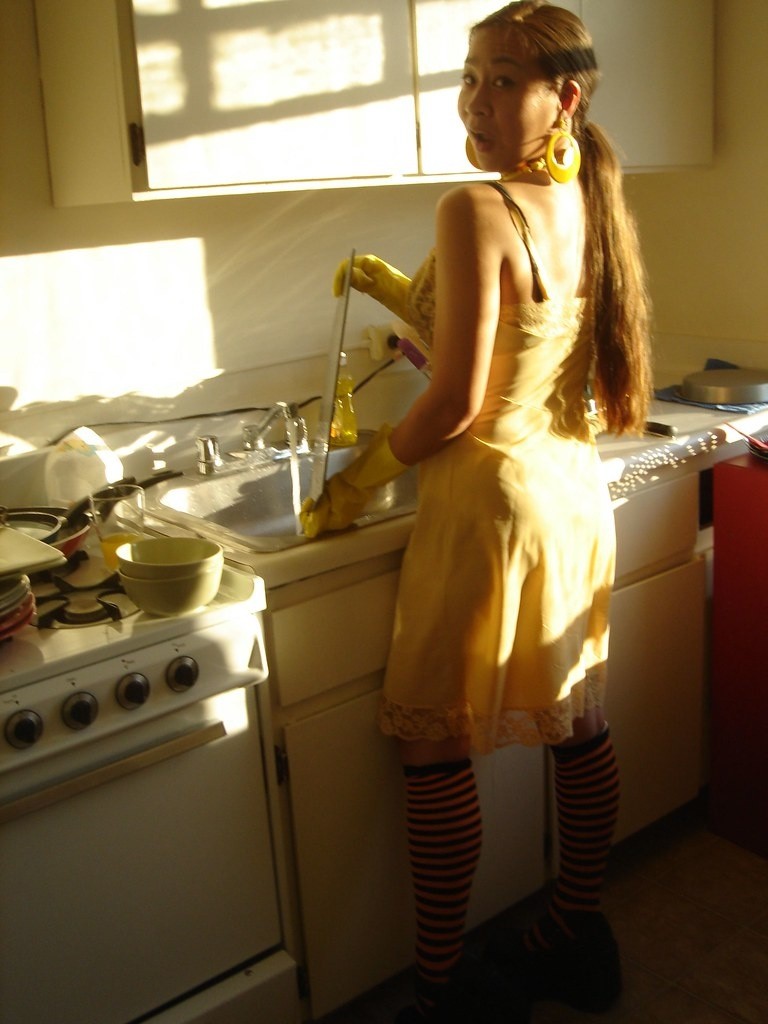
(0, 495), (271, 774)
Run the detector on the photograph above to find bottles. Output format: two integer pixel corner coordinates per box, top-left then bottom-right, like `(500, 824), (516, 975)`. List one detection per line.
(328, 351), (360, 447)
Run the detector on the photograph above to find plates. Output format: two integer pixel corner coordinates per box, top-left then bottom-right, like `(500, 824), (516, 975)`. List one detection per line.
(0, 521), (69, 642)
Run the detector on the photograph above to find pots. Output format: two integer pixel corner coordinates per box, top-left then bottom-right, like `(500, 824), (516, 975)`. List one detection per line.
(0, 471), (185, 568)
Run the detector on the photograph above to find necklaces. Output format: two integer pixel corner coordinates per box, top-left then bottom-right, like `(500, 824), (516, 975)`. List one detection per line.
(496, 155), (562, 181)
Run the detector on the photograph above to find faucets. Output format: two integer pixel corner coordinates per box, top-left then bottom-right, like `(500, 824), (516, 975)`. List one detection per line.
(241, 397), (298, 452)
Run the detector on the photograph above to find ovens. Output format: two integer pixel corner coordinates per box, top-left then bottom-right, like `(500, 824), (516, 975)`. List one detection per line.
(0, 681), (309, 1024)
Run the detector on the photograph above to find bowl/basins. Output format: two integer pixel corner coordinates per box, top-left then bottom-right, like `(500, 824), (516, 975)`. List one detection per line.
(116, 537), (224, 617)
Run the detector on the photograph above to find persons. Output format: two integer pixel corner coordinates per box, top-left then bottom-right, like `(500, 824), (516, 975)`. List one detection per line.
(301, 0), (656, 1023)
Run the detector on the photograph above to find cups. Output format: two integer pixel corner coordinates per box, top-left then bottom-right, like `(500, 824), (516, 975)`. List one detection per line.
(88, 484), (146, 575)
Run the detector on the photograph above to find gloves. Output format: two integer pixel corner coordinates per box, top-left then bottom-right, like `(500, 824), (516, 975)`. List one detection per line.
(298, 434), (414, 541)
(333, 254), (414, 329)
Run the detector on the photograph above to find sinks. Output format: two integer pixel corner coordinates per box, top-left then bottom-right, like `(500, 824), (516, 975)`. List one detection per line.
(138, 430), (421, 554)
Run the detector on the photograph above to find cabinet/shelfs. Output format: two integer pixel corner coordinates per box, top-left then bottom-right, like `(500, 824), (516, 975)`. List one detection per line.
(111, 394), (768, 1024)
(33, 0), (719, 210)
